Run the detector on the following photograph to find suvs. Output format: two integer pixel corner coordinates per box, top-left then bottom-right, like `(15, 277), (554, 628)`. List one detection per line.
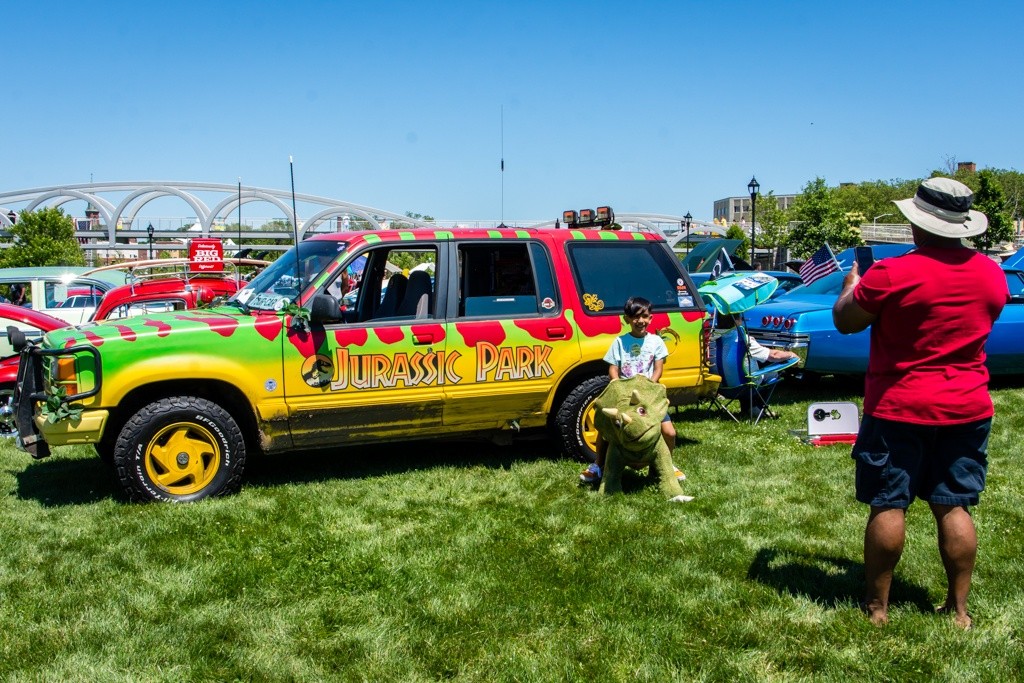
(6, 206), (722, 506)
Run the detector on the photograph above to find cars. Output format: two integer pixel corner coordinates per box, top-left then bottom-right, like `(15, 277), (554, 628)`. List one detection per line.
(0, 256), (437, 487)
(677, 245), (1024, 398)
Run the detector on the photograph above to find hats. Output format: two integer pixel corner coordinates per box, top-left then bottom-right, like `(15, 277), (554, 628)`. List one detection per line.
(890, 177), (988, 238)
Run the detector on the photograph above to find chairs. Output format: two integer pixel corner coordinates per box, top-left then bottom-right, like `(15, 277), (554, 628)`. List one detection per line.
(708, 325), (800, 425)
(380, 273), (409, 317)
(407, 271), (433, 315)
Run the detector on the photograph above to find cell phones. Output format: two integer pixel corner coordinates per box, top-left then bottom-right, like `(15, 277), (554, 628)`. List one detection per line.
(855, 247), (874, 278)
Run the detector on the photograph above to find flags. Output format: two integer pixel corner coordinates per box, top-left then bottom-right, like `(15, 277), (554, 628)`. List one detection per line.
(798, 245), (837, 287)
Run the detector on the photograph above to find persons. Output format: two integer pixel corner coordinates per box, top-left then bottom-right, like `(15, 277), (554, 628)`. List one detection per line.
(580, 296), (687, 481)
(753, 261), (761, 271)
(732, 219), (736, 225)
(340, 271), (355, 298)
(717, 309), (800, 417)
(402, 266), (409, 278)
(833, 177), (1010, 631)
(10, 283), (26, 306)
(995, 243), (1017, 262)
(739, 217), (745, 230)
(251, 266), (274, 292)
(714, 216), (727, 226)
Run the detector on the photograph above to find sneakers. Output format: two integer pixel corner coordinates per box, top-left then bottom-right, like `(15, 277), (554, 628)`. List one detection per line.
(672, 464), (687, 480)
(580, 463), (602, 482)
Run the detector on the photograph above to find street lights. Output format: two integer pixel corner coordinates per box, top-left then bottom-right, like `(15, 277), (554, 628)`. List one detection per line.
(146, 223), (154, 279)
(746, 174), (761, 270)
(874, 214), (894, 240)
(682, 210), (693, 255)
